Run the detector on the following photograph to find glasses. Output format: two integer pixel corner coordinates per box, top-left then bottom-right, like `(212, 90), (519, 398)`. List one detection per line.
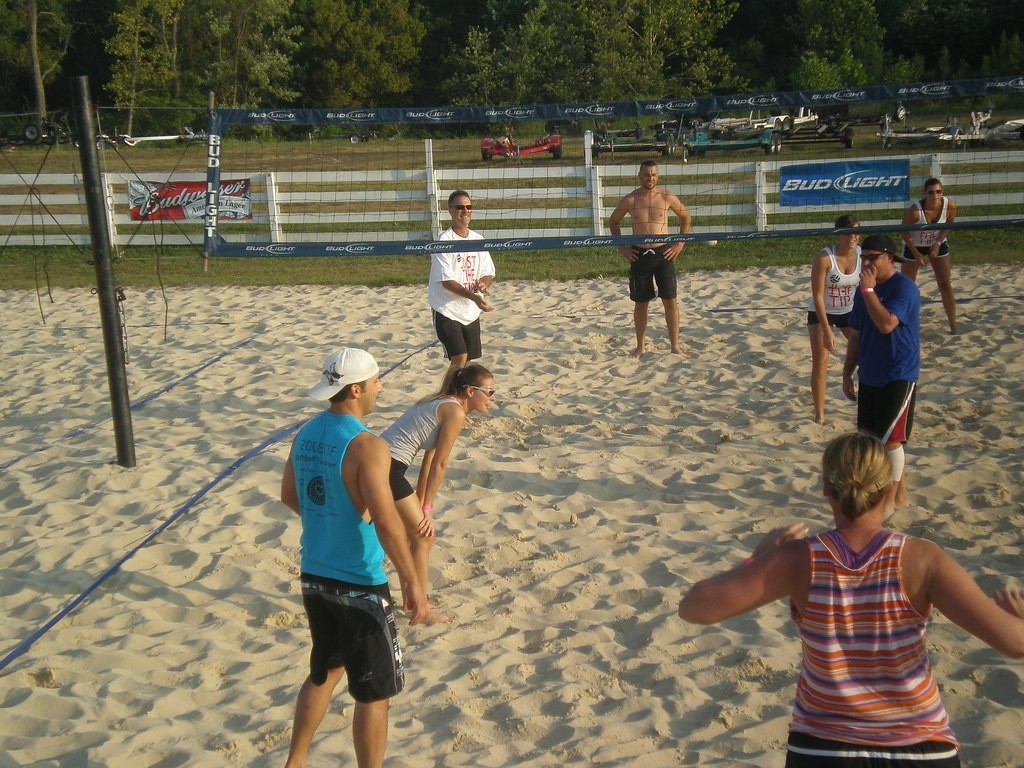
(450, 205), (473, 210)
(462, 384), (495, 397)
(860, 252), (885, 262)
(925, 189), (943, 195)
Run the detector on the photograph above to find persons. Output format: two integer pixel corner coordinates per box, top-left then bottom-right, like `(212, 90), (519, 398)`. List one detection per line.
(609, 161), (693, 359)
(427, 190), (495, 370)
(281, 347), (425, 768)
(379, 364), (496, 624)
(901, 178), (961, 336)
(807, 215), (862, 425)
(679, 432), (1024, 768)
(843, 235), (920, 521)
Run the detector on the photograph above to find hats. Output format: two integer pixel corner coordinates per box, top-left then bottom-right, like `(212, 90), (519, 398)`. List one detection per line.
(307, 348), (379, 401)
(861, 235), (909, 262)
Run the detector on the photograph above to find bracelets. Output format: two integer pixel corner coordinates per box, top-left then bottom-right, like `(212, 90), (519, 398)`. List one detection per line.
(937, 240), (941, 246)
(676, 242), (686, 246)
(422, 504), (433, 512)
(861, 288), (875, 294)
